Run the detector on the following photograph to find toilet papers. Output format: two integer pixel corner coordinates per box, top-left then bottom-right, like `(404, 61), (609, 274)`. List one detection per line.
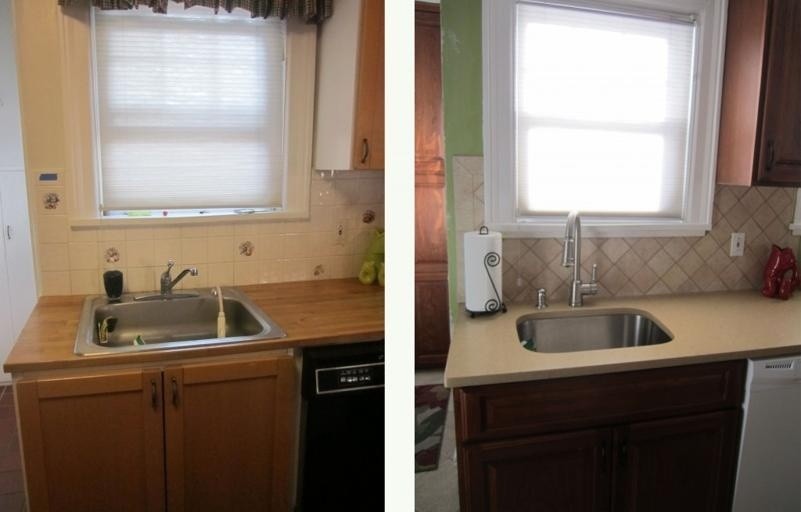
(463, 231), (503, 314)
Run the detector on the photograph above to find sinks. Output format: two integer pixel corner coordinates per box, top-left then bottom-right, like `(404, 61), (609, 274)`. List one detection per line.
(72, 286), (289, 357)
(515, 306), (675, 352)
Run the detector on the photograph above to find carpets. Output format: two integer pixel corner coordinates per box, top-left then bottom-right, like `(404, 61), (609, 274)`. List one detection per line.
(415, 384), (451, 473)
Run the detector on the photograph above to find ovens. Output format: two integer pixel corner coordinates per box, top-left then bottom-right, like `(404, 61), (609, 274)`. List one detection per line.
(298, 339), (386, 511)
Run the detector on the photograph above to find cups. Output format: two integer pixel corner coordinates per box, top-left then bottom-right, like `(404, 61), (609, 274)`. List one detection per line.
(102, 268), (124, 300)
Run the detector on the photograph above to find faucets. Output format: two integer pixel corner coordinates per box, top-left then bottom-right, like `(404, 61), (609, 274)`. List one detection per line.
(560, 211), (599, 308)
(160, 258), (199, 293)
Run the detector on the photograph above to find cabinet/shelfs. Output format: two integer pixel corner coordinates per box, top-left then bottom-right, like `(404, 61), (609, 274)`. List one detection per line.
(11, 349), (294, 512)
(716, 0), (801, 188)
(314, 0), (385, 172)
(454, 359), (747, 511)
